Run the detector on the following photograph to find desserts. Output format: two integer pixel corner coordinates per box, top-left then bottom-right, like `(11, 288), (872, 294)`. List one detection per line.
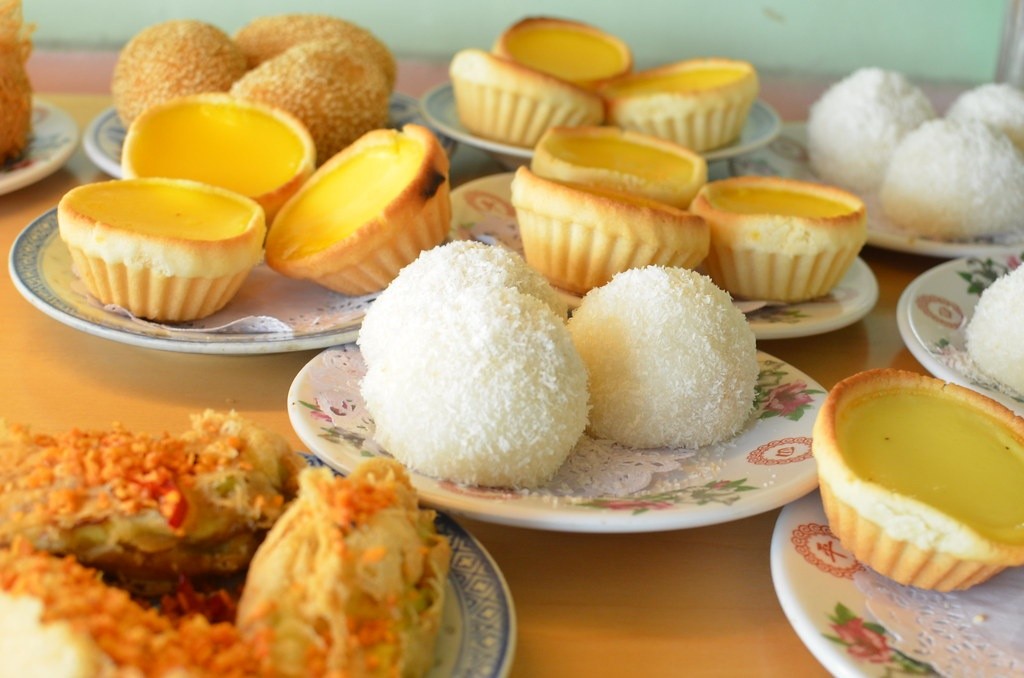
(0, 0), (1024, 678)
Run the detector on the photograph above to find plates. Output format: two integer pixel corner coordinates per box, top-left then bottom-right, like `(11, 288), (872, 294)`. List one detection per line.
(446, 173), (878, 336)
(421, 77), (782, 173)
(283, 453), (514, 678)
(9, 206), (362, 353)
(288, 346), (829, 533)
(734, 120), (1024, 259)
(772, 487), (1024, 678)
(0, 93), (80, 193)
(85, 92), (454, 180)
(898, 259), (1024, 422)
